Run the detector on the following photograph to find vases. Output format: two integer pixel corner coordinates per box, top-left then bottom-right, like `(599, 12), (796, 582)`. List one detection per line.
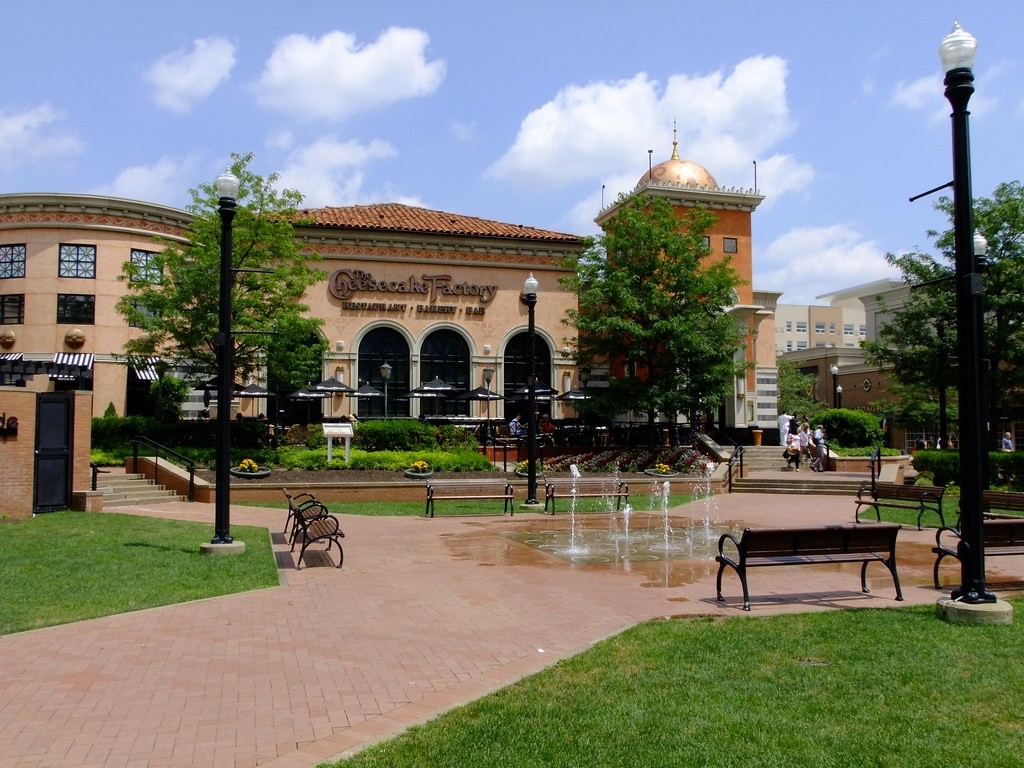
(516, 468), (542, 477)
(644, 469), (679, 478)
(230, 466), (271, 479)
(405, 469), (433, 479)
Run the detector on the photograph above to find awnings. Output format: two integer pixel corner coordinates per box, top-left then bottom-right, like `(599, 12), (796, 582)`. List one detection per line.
(130, 355), (161, 380)
(53, 352), (94, 369)
(0, 352), (24, 361)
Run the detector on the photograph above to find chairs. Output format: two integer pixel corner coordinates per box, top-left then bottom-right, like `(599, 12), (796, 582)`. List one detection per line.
(282, 487), (344, 569)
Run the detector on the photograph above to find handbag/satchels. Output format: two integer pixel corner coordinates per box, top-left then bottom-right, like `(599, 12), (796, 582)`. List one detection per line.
(812, 437), (820, 445)
(783, 445), (798, 458)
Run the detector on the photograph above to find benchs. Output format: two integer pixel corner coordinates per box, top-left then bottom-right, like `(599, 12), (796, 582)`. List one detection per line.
(854, 480), (946, 531)
(956, 490), (1024, 533)
(425, 484), (514, 517)
(545, 481), (629, 515)
(714, 523), (903, 611)
(931, 518), (1024, 589)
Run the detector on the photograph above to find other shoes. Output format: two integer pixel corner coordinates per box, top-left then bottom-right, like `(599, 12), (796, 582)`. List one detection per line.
(818, 470), (823, 472)
(797, 469), (800, 472)
(810, 467), (816, 472)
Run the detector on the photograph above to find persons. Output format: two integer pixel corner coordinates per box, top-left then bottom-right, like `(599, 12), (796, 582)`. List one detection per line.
(256, 413), (268, 422)
(1002, 432), (1014, 452)
(235, 413), (244, 421)
(777, 409), (825, 472)
(508, 411), (552, 435)
(916, 431), (954, 449)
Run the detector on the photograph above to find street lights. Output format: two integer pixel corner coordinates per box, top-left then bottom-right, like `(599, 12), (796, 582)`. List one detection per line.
(482, 364), (495, 446)
(209, 164), (241, 544)
(522, 271), (540, 508)
(937, 17), (995, 605)
(973, 226), (989, 513)
(831, 362), (843, 410)
(379, 360), (393, 419)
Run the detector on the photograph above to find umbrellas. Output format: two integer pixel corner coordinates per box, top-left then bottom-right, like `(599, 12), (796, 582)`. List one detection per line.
(452, 385), (506, 417)
(287, 376), (385, 424)
(403, 376), (466, 417)
(507, 377), (558, 418)
(194, 374), (275, 418)
(555, 385), (592, 418)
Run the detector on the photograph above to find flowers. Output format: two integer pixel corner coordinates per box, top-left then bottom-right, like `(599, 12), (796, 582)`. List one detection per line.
(411, 461), (427, 472)
(518, 460), (542, 474)
(239, 458), (258, 472)
(656, 463), (671, 474)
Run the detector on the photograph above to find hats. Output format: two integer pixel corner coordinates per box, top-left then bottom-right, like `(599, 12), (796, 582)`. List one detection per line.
(543, 414), (549, 418)
(818, 425), (823, 427)
